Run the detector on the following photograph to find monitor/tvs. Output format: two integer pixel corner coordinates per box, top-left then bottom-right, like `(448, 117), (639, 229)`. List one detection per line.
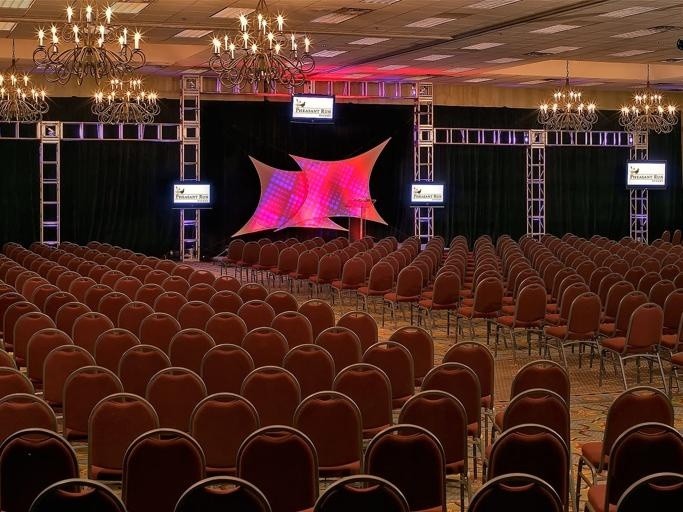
(624, 159), (671, 191)
(168, 180), (214, 210)
(406, 180), (449, 208)
(288, 93), (336, 125)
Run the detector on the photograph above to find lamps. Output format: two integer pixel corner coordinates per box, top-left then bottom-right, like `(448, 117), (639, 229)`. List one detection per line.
(206, 0), (317, 93)
(0, 35), (50, 125)
(618, 64), (681, 137)
(89, 77), (162, 127)
(34, 1), (145, 86)
(535, 59), (600, 135)
